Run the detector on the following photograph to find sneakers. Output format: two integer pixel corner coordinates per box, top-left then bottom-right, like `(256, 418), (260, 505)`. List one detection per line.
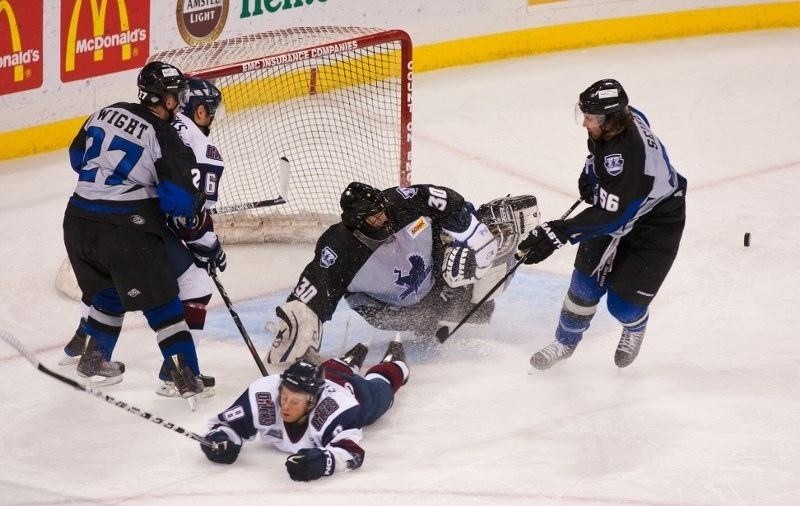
(344, 343), (367, 368)
(530, 340), (576, 369)
(159, 357), (215, 398)
(64, 319), (125, 376)
(614, 330), (643, 367)
(380, 340), (410, 385)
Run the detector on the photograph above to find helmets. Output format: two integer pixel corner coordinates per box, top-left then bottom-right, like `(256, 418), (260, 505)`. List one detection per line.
(182, 76), (221, 115)
(280, 359), (325, 394)
(574, 79), (627, 128)
(339, 182), (394, 233)
(137, 61), (190, 107)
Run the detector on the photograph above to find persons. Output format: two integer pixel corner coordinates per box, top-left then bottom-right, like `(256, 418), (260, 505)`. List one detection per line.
(62, 60), (228, 399)
(263, 180), (498, 375)
(61, 76), (225, 387)
(200, 340), (411, 483)
(514, 78), (688, 373)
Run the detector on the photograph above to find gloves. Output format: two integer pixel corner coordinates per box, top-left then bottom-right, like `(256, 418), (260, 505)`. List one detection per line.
(579, 156), (599, 204)
(514, 222), (566, 265)
(200, 421), (242, 464)
(190, 236), (226, 274)
(286, 448), (335, 481)
